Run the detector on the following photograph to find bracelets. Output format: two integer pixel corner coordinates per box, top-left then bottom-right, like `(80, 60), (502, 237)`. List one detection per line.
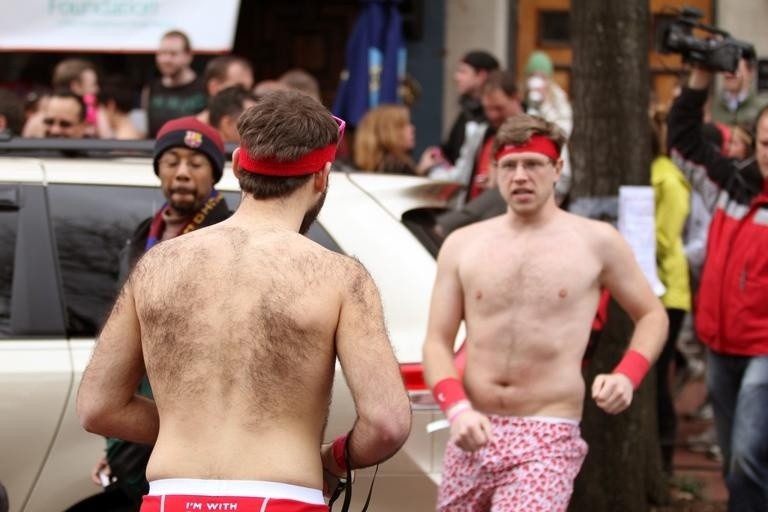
(330, 437), (354, 473)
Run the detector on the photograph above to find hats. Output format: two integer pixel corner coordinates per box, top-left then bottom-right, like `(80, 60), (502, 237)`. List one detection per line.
(154, 116), (224, 183)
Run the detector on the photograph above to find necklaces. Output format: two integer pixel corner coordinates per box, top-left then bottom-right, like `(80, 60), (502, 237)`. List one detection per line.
(162, 203), (194, 227)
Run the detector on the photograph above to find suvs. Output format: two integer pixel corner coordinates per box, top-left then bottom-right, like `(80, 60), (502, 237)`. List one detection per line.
(0, 132), (498, 512)
(344, 166), (511, 246)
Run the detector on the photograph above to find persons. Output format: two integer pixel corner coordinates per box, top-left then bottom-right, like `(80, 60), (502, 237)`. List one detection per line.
(416, 24), (767, 512)
(2, 23), (414, 175)
(82, 114), (234, 511)
(73, 86), (417, 512)
